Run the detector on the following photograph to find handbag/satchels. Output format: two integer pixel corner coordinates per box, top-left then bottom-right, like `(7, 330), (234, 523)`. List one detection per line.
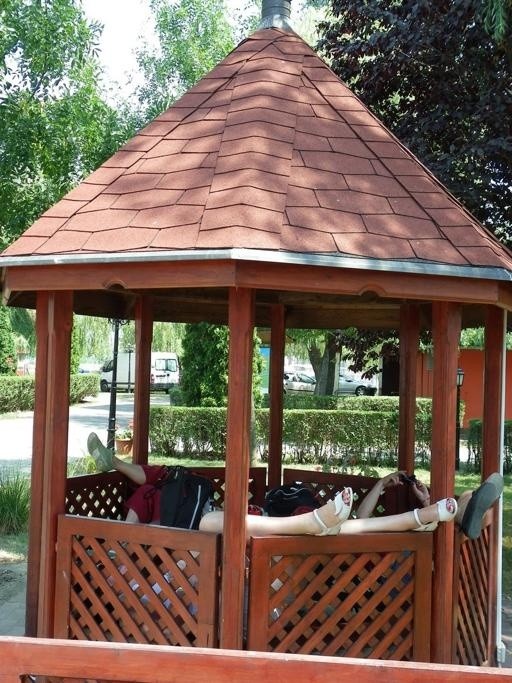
(158, 467), (211, 528)
(265, 483), (316, 517)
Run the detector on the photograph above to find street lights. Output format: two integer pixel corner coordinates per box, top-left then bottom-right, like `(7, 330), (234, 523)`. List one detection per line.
(123, 342), (135, 394)
(455, 366), (465, 471)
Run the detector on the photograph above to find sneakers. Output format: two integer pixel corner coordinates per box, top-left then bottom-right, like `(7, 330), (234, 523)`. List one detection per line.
(87, 431), (114, 474)
(462, 471), (505, 537)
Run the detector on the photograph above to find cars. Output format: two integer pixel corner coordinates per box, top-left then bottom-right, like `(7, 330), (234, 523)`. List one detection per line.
(282, 372), (377, 396)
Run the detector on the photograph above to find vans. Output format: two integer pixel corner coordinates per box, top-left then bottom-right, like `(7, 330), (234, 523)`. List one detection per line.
(100, 352), (180, 394)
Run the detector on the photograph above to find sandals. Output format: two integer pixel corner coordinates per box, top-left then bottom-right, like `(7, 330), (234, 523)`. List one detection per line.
(314, 486), (354, 538)
(410, 497), (458, 531)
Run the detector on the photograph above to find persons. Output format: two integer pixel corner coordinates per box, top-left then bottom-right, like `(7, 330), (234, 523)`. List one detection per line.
(88, 431), (506, 639)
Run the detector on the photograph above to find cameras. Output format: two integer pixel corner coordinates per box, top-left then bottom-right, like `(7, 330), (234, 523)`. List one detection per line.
(398, 474), (423, 489)
